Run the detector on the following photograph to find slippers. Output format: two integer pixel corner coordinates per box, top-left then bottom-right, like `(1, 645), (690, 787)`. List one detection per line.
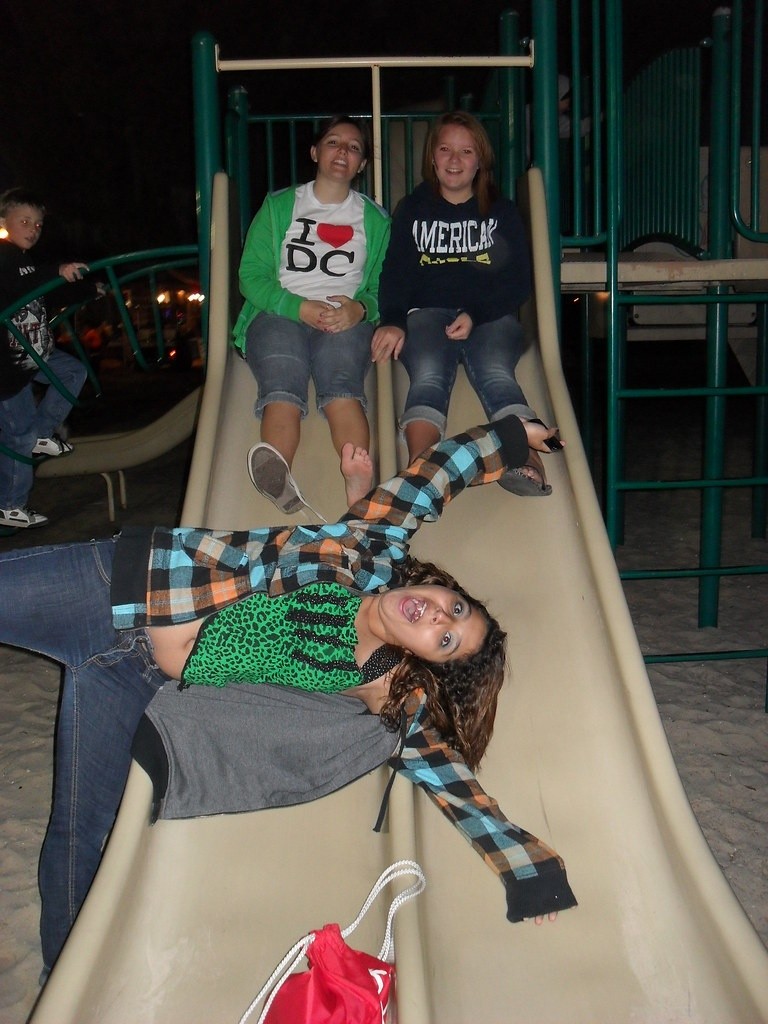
(496, 461), (553, 497)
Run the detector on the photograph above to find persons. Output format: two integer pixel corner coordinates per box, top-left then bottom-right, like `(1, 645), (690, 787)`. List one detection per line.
(233, 112), (391, 514)
(81, 320), (205, 378)
(557, 74), (602, 140)
(370, 108), (555, 497)
(0, 412), (580, 986)
(0, 187), (89, 528)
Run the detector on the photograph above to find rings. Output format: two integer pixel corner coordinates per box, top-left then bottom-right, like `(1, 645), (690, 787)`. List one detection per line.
(326, 304), (329, 309)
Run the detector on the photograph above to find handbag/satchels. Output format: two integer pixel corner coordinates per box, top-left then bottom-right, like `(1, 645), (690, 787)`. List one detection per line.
(236, 859), (426, 1024)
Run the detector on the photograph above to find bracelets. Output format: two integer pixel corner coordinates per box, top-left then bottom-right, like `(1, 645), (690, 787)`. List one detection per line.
(359, 301), (367, 322)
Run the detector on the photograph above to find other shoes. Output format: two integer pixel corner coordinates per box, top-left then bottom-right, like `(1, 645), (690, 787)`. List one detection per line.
(248, 440), (327, 523)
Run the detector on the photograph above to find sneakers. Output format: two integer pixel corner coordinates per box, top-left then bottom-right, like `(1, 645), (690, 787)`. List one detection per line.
(0, 507), (48, 527)
(32, 436), (75, 458)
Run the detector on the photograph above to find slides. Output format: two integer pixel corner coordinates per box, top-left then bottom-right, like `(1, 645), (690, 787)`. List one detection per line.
(30, 168), (767, 1024)
